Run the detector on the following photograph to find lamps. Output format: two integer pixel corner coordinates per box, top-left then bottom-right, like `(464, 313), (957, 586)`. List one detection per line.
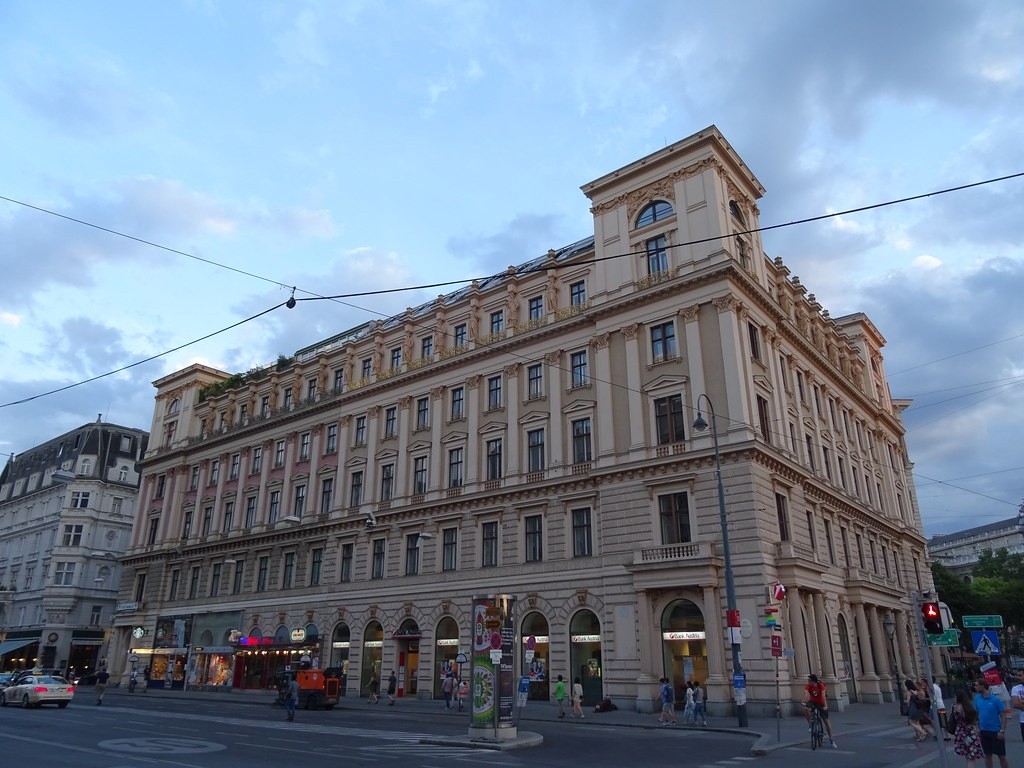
(286, 289), (297, 308)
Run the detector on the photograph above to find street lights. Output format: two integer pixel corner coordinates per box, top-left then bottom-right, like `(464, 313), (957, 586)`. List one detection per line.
(692, 392), (751, 728)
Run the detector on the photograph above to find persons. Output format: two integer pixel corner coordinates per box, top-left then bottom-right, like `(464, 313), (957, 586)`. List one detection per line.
(953, 678), (1008, 768)
(1011, 668), (1024, 742)
(551, 674), (565, 718)
(366, 673), (378, 704)
(656, 677), (676, 725)
(128, 668), (137, 693)
(69, 669), (76, 684)
(903, 676), (951, 741)
(683, 680), (707, 727)
(286, 681), (301, 721)
(96, 668), (109, 706)
(442, 671), (463, 710)
(387, 671), (397, 705)
(802, 674), (837, 748)
(569, 677), (584, 718)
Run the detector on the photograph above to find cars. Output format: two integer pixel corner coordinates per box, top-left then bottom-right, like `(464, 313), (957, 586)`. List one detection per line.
(0, 668), (109, 691)
(1, 674), (75, 710)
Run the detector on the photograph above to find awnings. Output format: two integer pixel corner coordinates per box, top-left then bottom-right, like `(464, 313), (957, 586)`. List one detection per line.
(0, 640), (37, 655)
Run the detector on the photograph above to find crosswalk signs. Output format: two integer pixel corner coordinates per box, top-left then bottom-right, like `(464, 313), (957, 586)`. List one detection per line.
(970, 630), (1002, 656)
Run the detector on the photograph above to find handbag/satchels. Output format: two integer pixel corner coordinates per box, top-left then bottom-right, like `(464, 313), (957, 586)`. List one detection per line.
(946, 704), (957, 735)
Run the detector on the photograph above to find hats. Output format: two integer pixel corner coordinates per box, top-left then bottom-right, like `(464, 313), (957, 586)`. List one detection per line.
(808, 674), (817, 682)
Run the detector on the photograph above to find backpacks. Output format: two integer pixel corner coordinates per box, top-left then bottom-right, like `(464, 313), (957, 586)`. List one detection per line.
(809, 680), (827, 697)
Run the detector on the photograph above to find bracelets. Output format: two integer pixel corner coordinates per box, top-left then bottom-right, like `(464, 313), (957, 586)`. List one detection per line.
(1000, 730), (1004, 732)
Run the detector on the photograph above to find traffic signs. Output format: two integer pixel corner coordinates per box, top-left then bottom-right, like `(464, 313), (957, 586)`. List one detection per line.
(923, 628), (960, 647)
(963, 615), (1004, 628)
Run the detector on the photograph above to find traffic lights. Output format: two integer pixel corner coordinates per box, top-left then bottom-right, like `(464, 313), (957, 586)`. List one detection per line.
(922, 601), (944, 634)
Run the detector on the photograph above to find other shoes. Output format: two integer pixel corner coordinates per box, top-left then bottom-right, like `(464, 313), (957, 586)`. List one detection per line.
(375, 700), (379, 704)
(580, 715), (584, 719)
(98, 699), (101, 703)
(389, 703), (393, 706)
(914, 732), (952, 742)
(563, 712), (565, 715)
(367, 702), (370, 703)
(808, 728), (812, 732)
(832, 741), (837, 748)
(658, 718), (707, 727)
(392, 700), (395, 704)
(97, 703), (101, 706)
(558, 716), (563, 718)
(574, 716), (578, 719)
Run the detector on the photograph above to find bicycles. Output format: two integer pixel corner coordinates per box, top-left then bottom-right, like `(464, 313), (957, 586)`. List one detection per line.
(801, 702), (827, 751)
